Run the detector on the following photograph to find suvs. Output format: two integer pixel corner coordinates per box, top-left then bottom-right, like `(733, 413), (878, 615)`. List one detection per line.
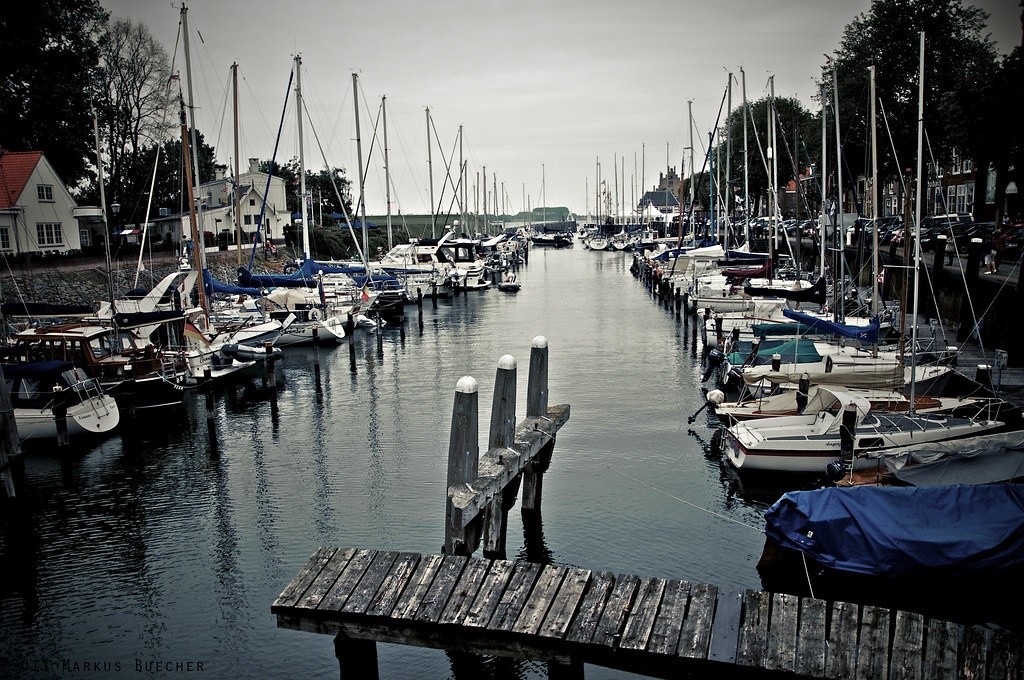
(892, 212), (975, 244)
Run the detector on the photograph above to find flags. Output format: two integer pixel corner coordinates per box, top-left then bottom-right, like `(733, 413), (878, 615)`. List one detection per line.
(830, 202), (835, 216)
(361, 286), (369, 302)
(735, 195), (743, 204)
(891, 231), (902, 244)
(184, 317), (210, 346)
(878, 269), (884, 283)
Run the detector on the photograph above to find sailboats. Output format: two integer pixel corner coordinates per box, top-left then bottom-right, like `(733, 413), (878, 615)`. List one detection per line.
(0, 107), (184, 446)
(689, 32), (1006, 476)
(579, 101), (697, 296)
(1, 2), (536, 400)
(537, 165), (575, 249)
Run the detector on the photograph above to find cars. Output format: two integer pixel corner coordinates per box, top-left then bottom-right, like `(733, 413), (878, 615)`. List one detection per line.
(919, 221), (1024, 258)
(707, 216), (818, 237)
(845, 217), (916, 243)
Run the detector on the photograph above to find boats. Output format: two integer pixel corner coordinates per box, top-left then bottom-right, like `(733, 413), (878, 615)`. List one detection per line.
(768, 479), (1024, 586)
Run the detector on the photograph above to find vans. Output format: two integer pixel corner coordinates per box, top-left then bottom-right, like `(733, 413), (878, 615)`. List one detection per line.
(818, 214), (867, 240)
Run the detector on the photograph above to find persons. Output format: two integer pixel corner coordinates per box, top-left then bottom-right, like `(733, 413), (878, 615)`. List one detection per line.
(266, 240), (277, 259)
(1003, 213), (1010, 224)
(977, 229), (1016, 275)
(253, 234), (258, 249)
(945, 231), (956, 266)
